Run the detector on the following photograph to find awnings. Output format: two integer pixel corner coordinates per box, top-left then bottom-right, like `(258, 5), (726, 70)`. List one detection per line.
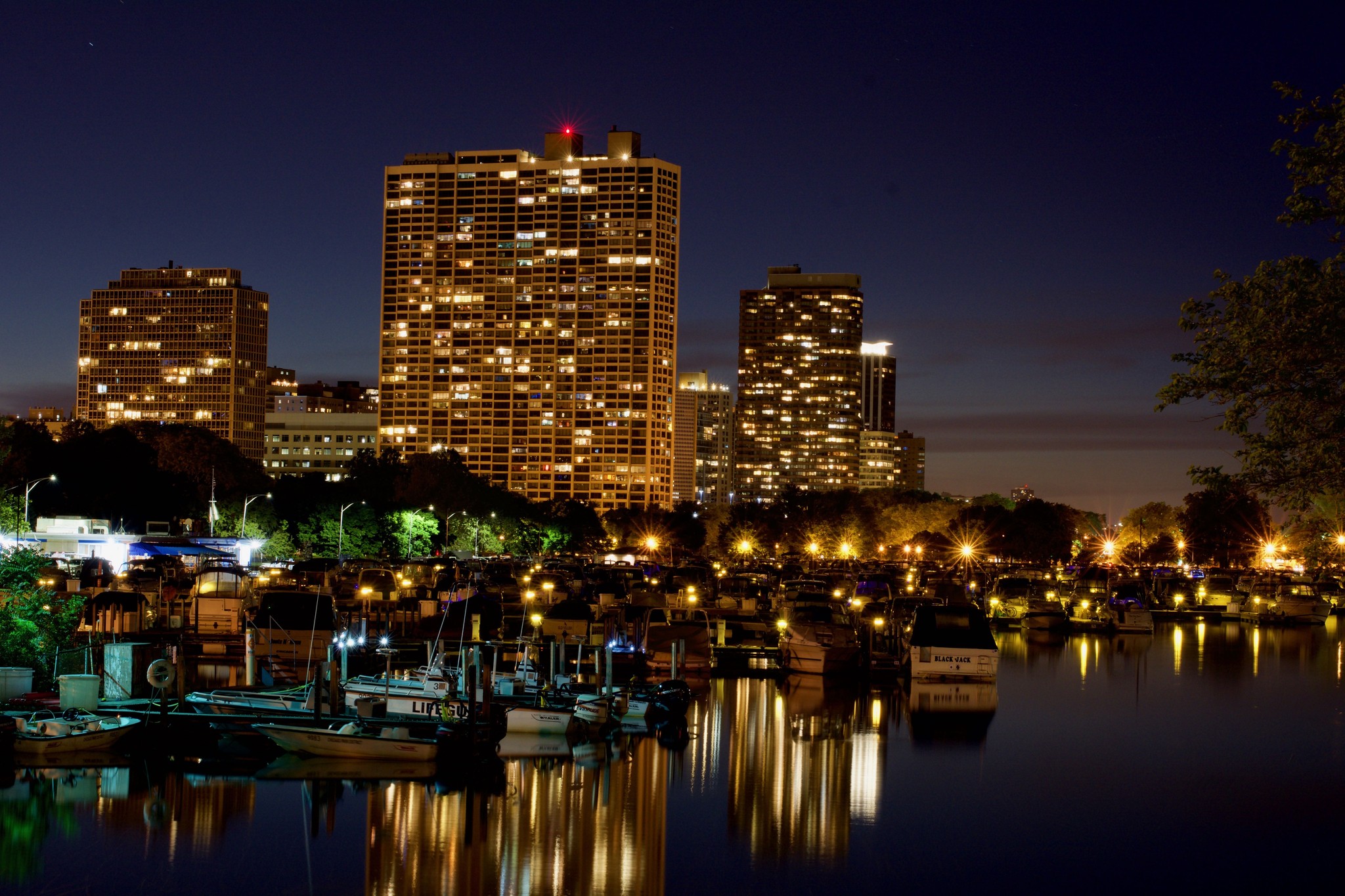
(129, 542), (237, 558)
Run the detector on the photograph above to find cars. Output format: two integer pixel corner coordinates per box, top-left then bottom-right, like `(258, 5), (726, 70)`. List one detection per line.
(81, 590), (162, 629)
(28, 552), (1345, 693)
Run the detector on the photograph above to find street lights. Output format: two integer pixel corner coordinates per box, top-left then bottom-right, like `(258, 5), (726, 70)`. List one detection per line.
(408, 505), (434, 558)
(446, 509), (466, 554)
(338, 501), (365, 559)
(25, 475), (56, 522)
(475, 512), (496, 556)
(241, 492), (272, 538)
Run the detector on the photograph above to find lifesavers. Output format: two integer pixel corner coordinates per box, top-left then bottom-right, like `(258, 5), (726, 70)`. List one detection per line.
(147, 659), (175, 689)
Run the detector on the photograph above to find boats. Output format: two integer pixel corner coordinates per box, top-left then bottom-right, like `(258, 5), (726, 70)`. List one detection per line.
(500, 686), (664, 734)
(250, 713), (439, 762)
(338, 582), (484, 725)
(183, 681), (314, 722)
(9, 708), (141, 754)
(7, 751), (132, 778)
